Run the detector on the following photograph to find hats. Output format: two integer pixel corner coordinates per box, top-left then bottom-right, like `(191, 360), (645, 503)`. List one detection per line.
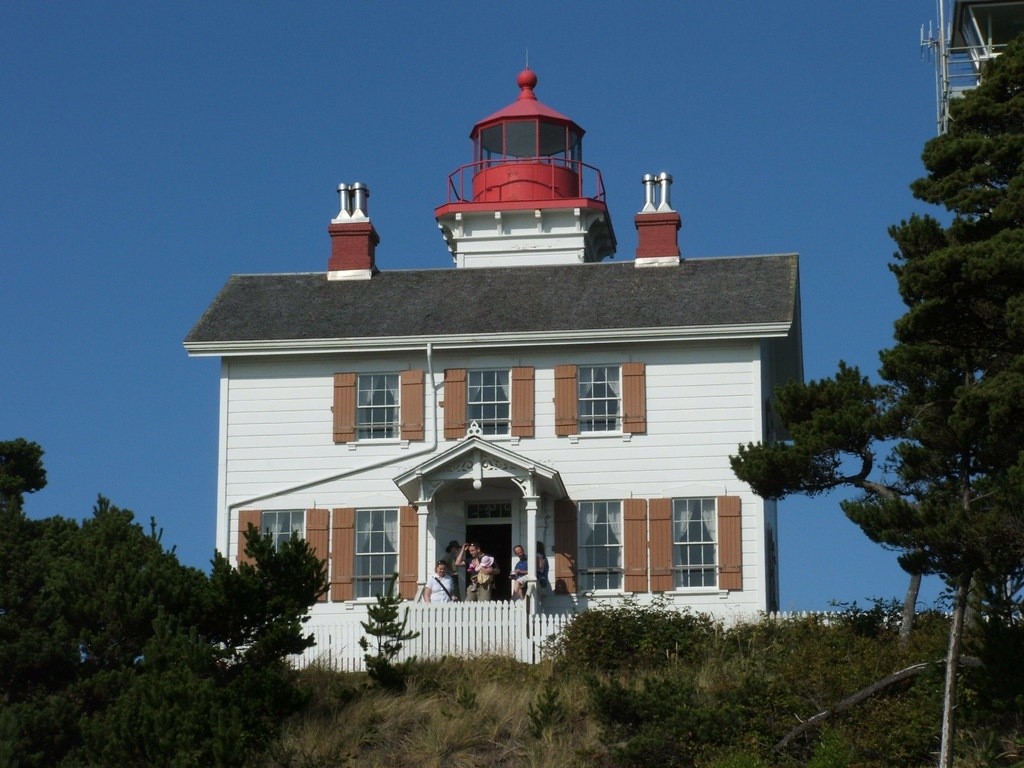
(480, 556), (494, 567)
(446, 540), (462, 553)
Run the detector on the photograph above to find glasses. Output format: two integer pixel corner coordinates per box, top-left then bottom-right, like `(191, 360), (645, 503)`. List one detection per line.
(438, 561), (445, 564)
(470, 543), (474, 546)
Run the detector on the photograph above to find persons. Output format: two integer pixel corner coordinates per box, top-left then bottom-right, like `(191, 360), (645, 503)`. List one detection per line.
(442, 540), (549, 603)
(423, 560), (458, 603)
